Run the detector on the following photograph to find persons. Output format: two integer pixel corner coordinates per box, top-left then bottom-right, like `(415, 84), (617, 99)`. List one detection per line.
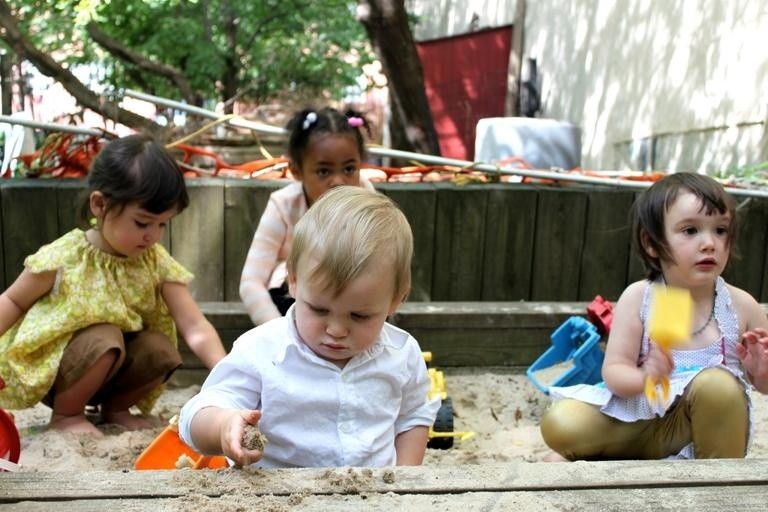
(236, 101), (382, 332)
(0, 133), (227, 441)
(538, 168), (768, 463)
(175, 181), (446, 473)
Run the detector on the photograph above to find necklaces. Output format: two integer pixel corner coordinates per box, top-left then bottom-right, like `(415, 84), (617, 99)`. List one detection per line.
(689, 283), (719, 339)
